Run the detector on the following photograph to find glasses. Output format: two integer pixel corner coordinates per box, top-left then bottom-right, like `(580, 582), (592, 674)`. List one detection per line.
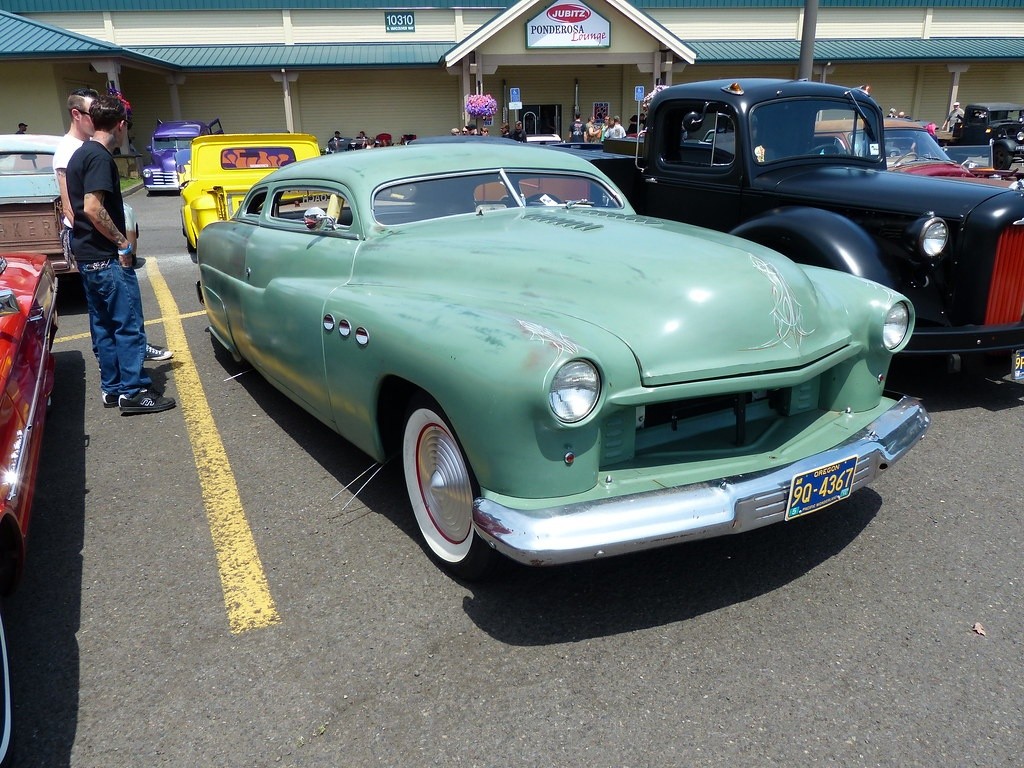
(119, 119), (133, 130)
(462, 131), (468, 133)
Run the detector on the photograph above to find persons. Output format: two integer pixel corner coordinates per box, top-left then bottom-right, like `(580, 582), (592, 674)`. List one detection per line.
(888, 106), (905, 120)
(597, 116), (613, 143)
(54, 86), (174, 372)
(626, 113), (645, 134)
(355, 130), (380, 149)
(499, 122), (512, 138)
(465, 124), (477, 134)
(15, 122), (28, 134)
(584, 114), (601, 143)
(450, 128), (460, 136)
(603, 115), (627, 139)
(66, 89), (177, 415)
(941, 101), (965, 133)
(462, 126), (471, 136)
(568, 112), (588, 143)
(328, 131), (344, 152)
(479, 126), (490, 137)
(860, 83), (871, 93)
(509, 121), (528, 143)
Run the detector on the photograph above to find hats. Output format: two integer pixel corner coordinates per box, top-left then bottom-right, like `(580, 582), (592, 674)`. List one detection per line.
(953, 102), (960, 105)
(18, 123), (28, 127)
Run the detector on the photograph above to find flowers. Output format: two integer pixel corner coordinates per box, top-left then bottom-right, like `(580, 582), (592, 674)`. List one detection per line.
(642, 85), (670, 120)
(466, 94), (498, 117)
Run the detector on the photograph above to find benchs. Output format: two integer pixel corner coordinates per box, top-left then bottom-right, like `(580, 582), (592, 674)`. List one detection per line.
(279, 206), (421, 226)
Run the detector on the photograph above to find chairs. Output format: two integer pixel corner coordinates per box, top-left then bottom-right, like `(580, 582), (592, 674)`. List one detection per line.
(402, 134), (416, 145)
(375, 133), (392, 147)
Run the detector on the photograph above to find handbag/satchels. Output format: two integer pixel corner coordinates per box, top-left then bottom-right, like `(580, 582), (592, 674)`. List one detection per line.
(596, 129), (602, 139)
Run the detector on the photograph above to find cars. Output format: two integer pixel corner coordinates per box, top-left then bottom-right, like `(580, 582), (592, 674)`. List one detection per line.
(547, 74), (1024, 407)
(176, 131), (322, 253)
(0, 249), (61, 613)
(138, 116), (226, 196)
(0, 133), (140, 284)
(190, 141), (935, 589)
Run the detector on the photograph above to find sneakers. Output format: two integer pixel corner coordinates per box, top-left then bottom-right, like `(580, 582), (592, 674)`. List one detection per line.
(119, 389), (175, 412)
(141, 346), (174, 361)
(101, 390), (119, 407)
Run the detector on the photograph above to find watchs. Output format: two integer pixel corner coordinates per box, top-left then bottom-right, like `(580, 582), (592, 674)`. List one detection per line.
(118, 242), (133, 256)
